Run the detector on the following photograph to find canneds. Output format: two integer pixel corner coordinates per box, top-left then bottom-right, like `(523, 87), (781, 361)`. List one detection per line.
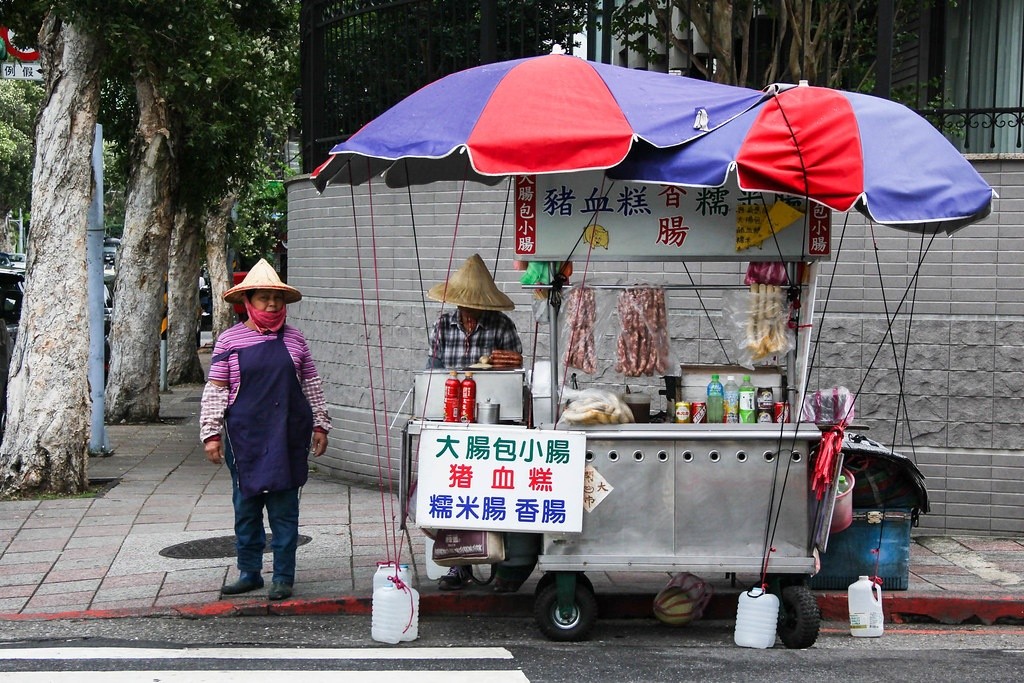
(690, 402), (708, 423)
(756, 387), (789, 423)
(675, 401), (691, 423)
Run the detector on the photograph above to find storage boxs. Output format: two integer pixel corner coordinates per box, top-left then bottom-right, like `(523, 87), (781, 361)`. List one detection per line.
(822, 509), (912, 587)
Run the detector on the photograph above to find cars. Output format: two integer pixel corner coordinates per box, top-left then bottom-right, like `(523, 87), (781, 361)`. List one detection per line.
(0, 238), (250, 371)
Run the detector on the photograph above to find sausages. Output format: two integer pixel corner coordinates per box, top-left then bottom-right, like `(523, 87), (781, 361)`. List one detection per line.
(563, 285), (668, 378)
(563, 399), (630, 425)
(747, 284), (786, 357)
(490, 350), (522, 366)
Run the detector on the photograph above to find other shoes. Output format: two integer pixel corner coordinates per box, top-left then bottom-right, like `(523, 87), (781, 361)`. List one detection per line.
(267, 580), (292, 600)
(221, 570), (264, 595)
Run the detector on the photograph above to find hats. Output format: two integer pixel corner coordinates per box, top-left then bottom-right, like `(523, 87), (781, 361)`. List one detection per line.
(426, 253), (515, 312)
(223, 258), (302, 304)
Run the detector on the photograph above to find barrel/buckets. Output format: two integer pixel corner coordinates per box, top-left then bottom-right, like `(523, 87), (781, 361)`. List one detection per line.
(830, 467), (856, 535)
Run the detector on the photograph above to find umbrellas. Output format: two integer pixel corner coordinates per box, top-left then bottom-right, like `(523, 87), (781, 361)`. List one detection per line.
(309, 47), (797, 432)
(608, 81), (996, 425)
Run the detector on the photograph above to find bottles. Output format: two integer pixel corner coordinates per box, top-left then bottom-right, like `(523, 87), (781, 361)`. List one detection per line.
(738, 375), (757, 423)
(461, 372), (476, 423)
(837, 474), (849, 495)
(723, 376), (739, 422)
(444, 370), (461, 422)
(425, 535), (451, 579)
(367, 561), (423, 645)
(732, 583), (782, 653)
(846, 572), (885, 643)
(706, 374), (724, 422)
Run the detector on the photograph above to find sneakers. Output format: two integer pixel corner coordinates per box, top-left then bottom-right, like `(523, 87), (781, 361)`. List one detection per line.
(494, 578), (518, 592)
(438, 565), (473, 590)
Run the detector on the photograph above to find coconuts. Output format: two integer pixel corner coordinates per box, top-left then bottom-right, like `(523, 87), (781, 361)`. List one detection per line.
(653, 571), (707, 625)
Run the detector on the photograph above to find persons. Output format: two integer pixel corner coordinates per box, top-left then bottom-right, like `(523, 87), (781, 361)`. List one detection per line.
(200, 258), (333, 601)
(429, 256), (525, 589)
